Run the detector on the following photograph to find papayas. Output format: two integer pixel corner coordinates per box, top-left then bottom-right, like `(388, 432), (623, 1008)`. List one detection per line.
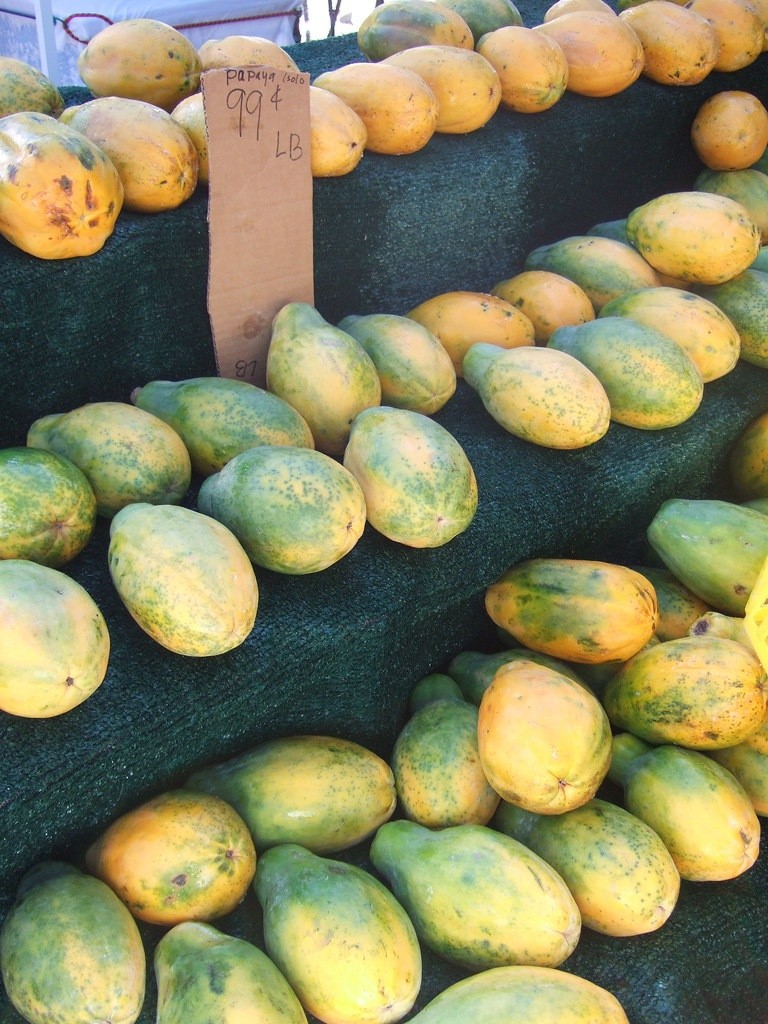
(1, 1), (768, 1024)
